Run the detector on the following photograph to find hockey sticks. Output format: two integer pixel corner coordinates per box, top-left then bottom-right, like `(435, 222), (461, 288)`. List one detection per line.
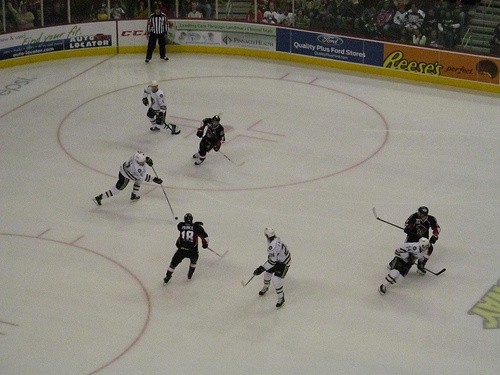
(151, 165), (174, 216)
(208, 247), (229, 257)
(373, 207), (405, 231)
(147, 103), (181, 135)
(411, 262), (446, 275)
(201, 134), (245, 167)
(241, 260), (269, 287)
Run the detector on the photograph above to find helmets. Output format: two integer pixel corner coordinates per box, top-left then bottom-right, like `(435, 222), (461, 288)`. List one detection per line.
(418, 207), (428, 215)
(136, 153), (146, 163)
(184, 213), (194, 222)
(419, 237), (431, 252)
(150, 80), (158, 87)
(212, 115), (220, 121)
(264, 227), (275, 237)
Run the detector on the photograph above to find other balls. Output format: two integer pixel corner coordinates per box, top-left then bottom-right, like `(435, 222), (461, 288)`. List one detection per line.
(174, 217), (178, 220)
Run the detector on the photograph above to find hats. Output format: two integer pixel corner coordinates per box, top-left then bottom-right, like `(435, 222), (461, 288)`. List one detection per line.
(154, 4), (160, 8)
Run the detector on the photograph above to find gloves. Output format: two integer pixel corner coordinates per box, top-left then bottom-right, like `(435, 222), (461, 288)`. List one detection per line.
(159, 112), (166, 120)
(254, 265), (265, 276)
(197, 127), (204, 137)
(202, 237), (209, 248)
(408, 257), (414, 264)
(430, 235), (438, 244)
(153, 177), (163, 184)
(146, 156), (153, 166)
(214, 144), (220, 151)
(404, 225), (411, 234)
(418, 262), (424, 269)
(143, 97), (149, 106)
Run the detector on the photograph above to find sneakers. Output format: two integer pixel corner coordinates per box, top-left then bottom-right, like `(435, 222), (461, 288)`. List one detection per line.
(193, 152), (203, 165)
(258, 286), (268, 295)
(92, 196), (102, 206)
(145, 58), (150, 64)
(150, 127), (160, 130)
(131, 192), (140, 200)
(188, 270), (193, 279)
(165, 271), (172, 283)
(160, 56), (168, 62)
(276, 297), (285, 310)
(378, 283), (385, 295)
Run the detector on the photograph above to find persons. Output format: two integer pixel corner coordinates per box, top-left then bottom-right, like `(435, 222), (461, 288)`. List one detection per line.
(245, 0), (480, 49)
(194, 115), (226, 166)
(378, 237), (431, 296)
(95, 150), (163, 206)
(89, 0), (214, 20)
(163, 213), (209, 284)
(145, 7), (169, 62)
(4, 0), (68, 30)
(254, 227), (291, 307)
(142, 80), (168, 132)
(404, 206), (441, 273)
(491, 23), (500, 57)
